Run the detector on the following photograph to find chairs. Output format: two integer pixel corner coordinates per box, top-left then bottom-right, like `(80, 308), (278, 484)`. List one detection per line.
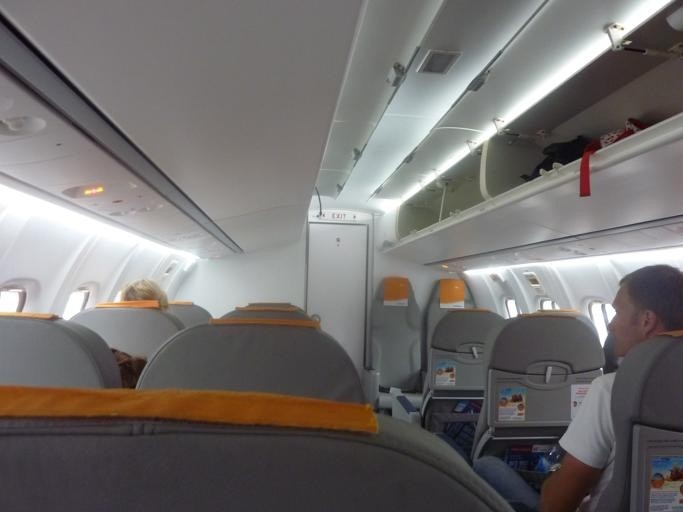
(584, 328), (683, 511)
(68, 299), (187, 366)
(1, 310), (125, 389)
(468, 307), (605, 512)
(362, 275), (424, 415)
(132, 318), (370, 408)
(1, 382), (514, 511)
(419, 306), (505, 466)
(168, 300), (214, 330)
(424, 275), (478, 386)
(217, 299), (323, 329)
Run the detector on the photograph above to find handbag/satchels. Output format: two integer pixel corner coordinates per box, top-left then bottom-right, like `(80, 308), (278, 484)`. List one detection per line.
(520, 119), (647, 181)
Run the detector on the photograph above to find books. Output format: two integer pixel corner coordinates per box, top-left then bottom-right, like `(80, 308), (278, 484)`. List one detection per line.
(443, 398), (483, 454)
(502, 442), (559, 475)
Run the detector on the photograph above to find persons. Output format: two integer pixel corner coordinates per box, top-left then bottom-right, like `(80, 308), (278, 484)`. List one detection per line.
(120, 278), (169, 314)
(472, 261), (683, 510)
